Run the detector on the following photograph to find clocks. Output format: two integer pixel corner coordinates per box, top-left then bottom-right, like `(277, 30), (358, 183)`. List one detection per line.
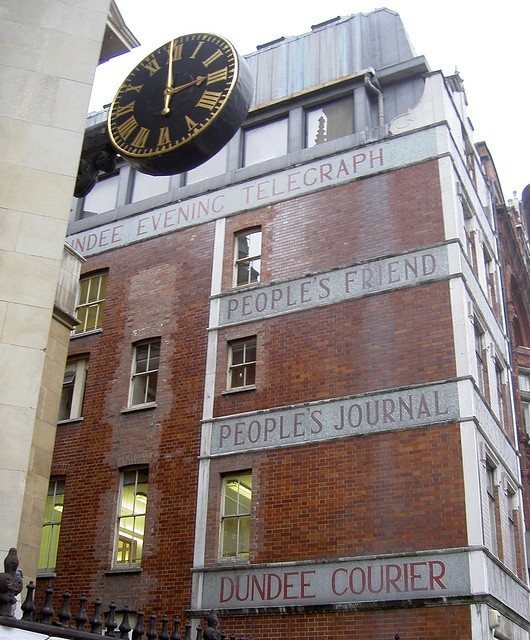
(107, 33), (254, 176)
